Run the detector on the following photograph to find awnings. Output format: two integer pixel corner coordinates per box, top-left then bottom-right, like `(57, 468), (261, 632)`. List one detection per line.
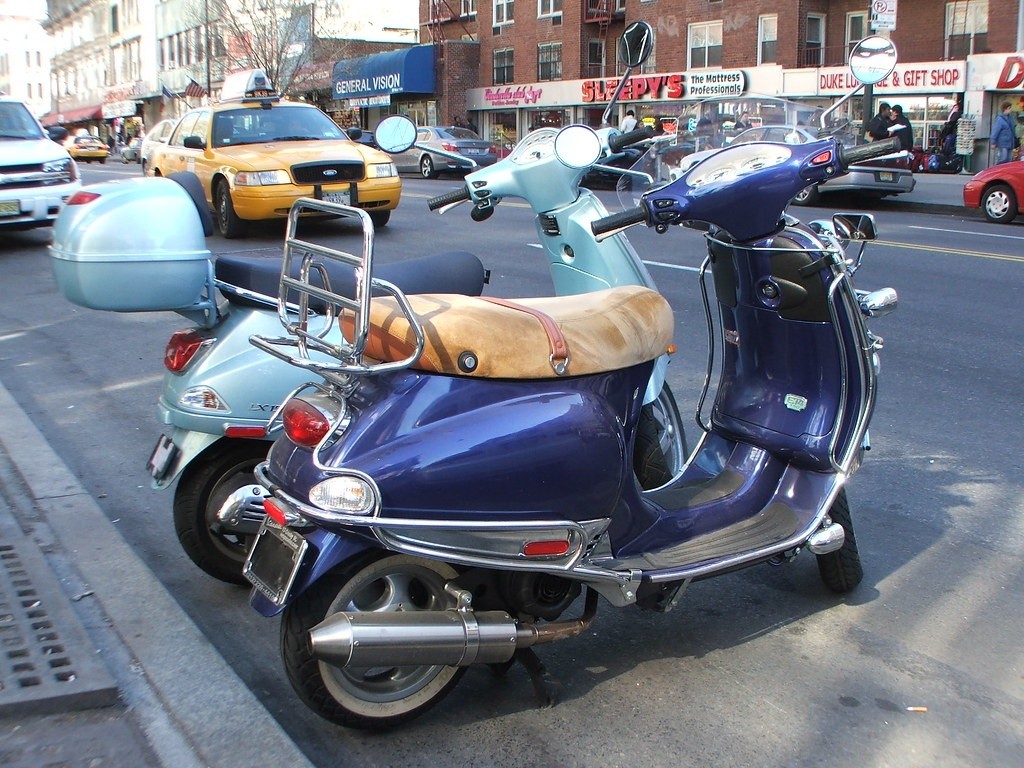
(333, 44), (436, 100)
(220, 69), (273, 99)
(41, 105), (102, 128)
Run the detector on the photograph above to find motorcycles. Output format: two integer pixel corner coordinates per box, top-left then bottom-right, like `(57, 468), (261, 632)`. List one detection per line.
(242, 34), (903, 736)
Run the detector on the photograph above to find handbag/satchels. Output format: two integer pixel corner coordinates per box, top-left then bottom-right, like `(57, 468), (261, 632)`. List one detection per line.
(1014, 136), (1020, 149)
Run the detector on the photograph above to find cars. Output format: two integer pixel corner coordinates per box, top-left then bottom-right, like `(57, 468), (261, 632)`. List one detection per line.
(65, 129), (111, 164)
(343, 126), (382, 151)
(120, 135), (145, 164)
(1, 92), (82, 230)
(146, 68), (404, 240)
(45, 126), (72, 146)
(963, 155), (1024, 224)
(679, 123), (917, 207)
(389, 126), (498, 180)
(141, 119), (179, 171)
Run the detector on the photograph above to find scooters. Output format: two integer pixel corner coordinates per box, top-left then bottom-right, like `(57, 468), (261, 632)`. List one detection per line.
(45, 21), (662, 592)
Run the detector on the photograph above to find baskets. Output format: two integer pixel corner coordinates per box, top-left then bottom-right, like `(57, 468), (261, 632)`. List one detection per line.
(955, 118), (976, 155)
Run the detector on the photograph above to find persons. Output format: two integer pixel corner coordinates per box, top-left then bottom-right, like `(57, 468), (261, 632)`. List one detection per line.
(696, 110), (713, 127)
(734, 111), (754, 130)
(808, 105), (824, 127)
(466, 117), (478, 135)
(453, 116), (465, 128)
(528, 126), (534, 132)
(990, 102), (1016, 165)
(869, 103), (913, 153)
(620, 110), (638, 134)
(940, 103), (961, 166)
(105, 133), (141, 156)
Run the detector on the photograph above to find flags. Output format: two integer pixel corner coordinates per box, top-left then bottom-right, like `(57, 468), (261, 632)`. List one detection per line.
(161, 85), (175, 99)
(184, 81), (206, 99)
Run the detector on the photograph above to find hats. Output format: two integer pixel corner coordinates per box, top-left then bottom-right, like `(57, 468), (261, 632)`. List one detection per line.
(891, 105), (902, 111)
